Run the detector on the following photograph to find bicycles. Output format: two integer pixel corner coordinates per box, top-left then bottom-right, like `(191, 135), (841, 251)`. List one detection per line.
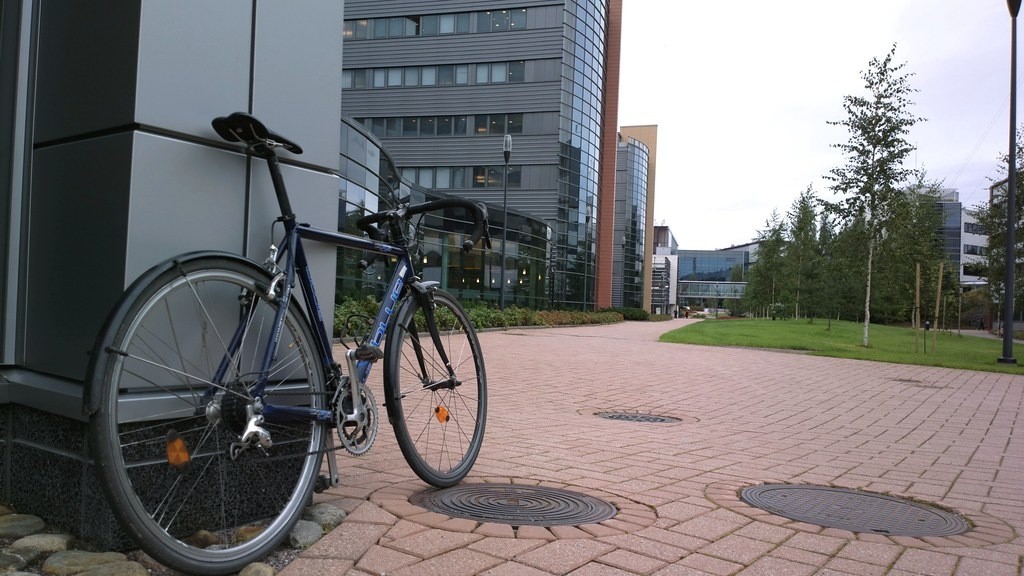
(80, 111), (493, 576)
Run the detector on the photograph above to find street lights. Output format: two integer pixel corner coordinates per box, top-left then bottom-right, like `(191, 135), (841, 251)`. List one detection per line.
(667, 278), (669, 314)
(620, 237), (626, 311)
(661, 272), (664, 315)
(715, 282), (719, 320)
(652, 266), (656, 315)
(772, 277), (776, 310)
(997, 0), (1022, 364)
(583, 206), (592, 313)
(499, 134), (513, 311)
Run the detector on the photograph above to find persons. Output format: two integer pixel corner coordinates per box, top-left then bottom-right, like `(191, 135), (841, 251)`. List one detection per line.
(673, 309), (678, 318)
(685, 310), (689, 319)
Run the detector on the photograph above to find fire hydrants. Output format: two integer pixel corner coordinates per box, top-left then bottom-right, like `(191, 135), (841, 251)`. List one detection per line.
(772, 311), (776, 320)
